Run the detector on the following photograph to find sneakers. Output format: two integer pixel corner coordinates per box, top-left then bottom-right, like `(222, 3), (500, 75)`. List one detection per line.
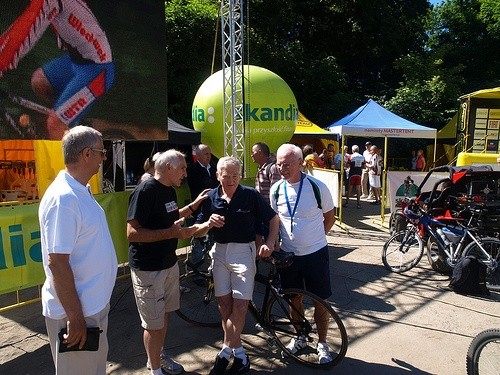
(317, 342), (333, 364)
(280, 335), (309, 357)
(147, 346), (184, 375)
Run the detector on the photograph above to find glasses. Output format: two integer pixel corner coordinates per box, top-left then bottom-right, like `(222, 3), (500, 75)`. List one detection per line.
(276, 158), (299, 168)
(79, 146), (107, 157)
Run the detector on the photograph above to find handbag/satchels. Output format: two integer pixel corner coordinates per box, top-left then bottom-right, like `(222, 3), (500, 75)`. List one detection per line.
(58, 327), (103, 353)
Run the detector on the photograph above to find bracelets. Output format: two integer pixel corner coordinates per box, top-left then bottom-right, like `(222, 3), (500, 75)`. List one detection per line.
(189, 204), (195, 213)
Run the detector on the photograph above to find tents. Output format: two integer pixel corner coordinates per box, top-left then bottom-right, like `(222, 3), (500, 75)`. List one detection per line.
(325, 98), (438, 225)
(168, 119), (200, 138)
(296, 110), (340, 156)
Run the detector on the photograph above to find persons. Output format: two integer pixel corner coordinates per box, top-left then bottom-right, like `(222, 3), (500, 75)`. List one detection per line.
(183, 144), (218, 274)
(268, 144), (336, 365)
(435, 181), (450, 198)
(415, 150), (425, 172)
(190, 155), (280, 375)
(0, 0), (115, 139)
(139, 156), (155, 186)
(125, 149), (213, 375)
(38, 126), (118, 375)
(411, 151), (417, 171)
(251, 143), (281, 263)
(268, 151), (276, 163)
(301, 142), (383, 209)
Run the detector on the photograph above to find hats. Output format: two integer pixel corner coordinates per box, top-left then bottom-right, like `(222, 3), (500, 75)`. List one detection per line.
(364, 141), (372, 146)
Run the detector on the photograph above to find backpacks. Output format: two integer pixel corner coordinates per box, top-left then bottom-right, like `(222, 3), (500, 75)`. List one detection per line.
(448, 255), (490, 296)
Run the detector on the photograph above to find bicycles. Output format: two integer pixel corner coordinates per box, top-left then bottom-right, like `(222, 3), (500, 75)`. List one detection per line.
(175, 233), (349, 370)
(1, 83), (137, 141)
(380, 199), (500, 293)
(465, 327), (500, 375)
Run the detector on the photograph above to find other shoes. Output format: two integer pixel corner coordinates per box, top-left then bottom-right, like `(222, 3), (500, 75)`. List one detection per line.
(343, 203), (349, 207)
(226, 353), (250, 375)
(372, 200), (381, 205)
(208, 352), (230, 375)
(357, 205), (361, 209)
